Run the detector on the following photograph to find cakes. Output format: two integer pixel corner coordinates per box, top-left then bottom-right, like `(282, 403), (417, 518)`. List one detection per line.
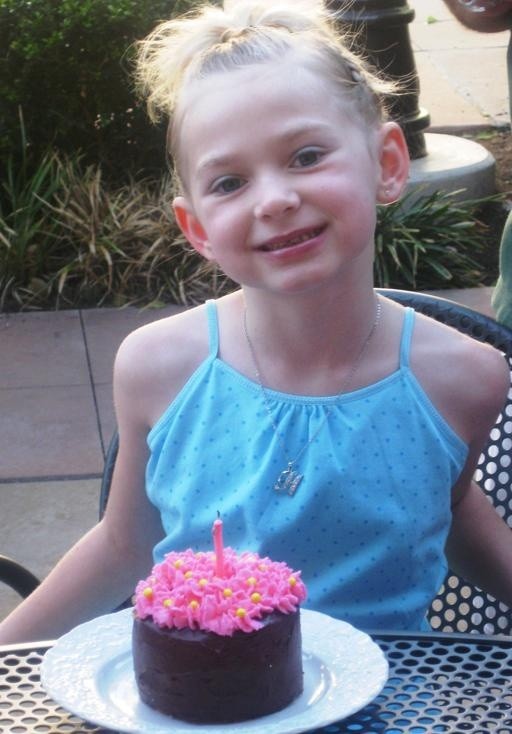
(134, 547), (306, 726)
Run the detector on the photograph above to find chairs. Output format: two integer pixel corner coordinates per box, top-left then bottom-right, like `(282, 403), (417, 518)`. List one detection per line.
(2, 287), (512, 640)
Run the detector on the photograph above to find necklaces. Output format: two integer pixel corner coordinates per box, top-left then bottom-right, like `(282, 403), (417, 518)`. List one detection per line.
(242, 293), (381, 497)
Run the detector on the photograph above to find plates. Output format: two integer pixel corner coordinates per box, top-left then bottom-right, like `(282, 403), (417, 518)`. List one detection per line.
(40, 603), (389, 728)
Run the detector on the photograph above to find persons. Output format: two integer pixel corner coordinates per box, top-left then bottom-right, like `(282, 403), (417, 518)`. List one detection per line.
(0, 5), (512, 733)
